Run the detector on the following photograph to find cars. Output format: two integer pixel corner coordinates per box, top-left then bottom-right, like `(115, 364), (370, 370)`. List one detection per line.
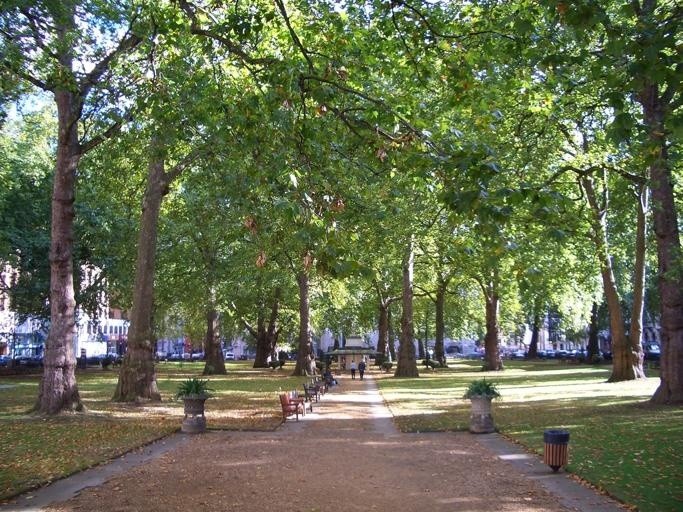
(456, 341), (664, 365)
(0, 346), (238, 367)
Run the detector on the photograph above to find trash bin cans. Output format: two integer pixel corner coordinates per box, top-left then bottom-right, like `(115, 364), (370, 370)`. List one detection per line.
(545, 430), (569, 472)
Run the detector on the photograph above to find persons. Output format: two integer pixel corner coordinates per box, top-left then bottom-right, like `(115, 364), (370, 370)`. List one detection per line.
(351, 360), (356, 379)
(358, 359), (365, 380)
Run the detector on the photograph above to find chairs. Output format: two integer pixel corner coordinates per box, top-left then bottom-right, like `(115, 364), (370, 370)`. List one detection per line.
(280, 372), (330, 422)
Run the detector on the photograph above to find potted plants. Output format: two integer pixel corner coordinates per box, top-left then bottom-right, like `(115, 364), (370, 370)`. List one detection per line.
(174, 376), (215, 414)
(463, 376), (502, 416)
